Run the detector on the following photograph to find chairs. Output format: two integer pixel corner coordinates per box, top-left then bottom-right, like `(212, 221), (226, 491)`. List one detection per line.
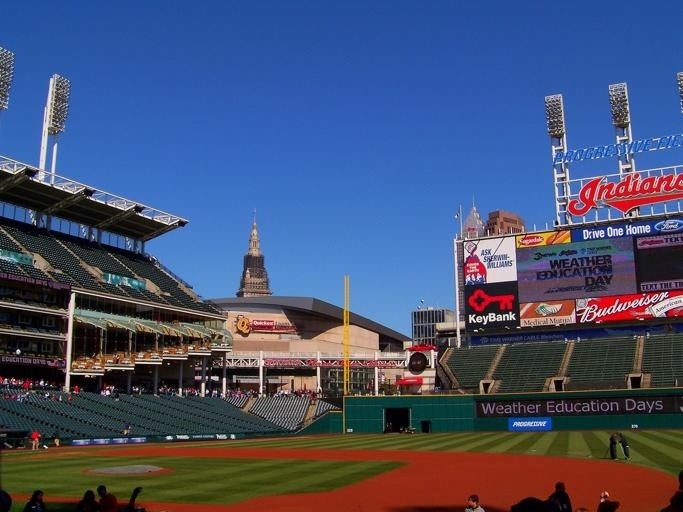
(1, 216), (683, 440)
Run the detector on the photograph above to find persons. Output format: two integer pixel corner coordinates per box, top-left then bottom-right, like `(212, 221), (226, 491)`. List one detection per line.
(461, 490), (489, 511)
(596, 490), (620, 511)
(616, 432), (633, 461)
(1, 374), (324, 404)
(0, 483), (149, 512)
(30, 428), (41, 450)
(608, 431), (620, 461)
(660, 470), (683, 512)
(71, 338), (207, 369)
(123, 419), (129, 436)
(549, 481), (572, 511)
